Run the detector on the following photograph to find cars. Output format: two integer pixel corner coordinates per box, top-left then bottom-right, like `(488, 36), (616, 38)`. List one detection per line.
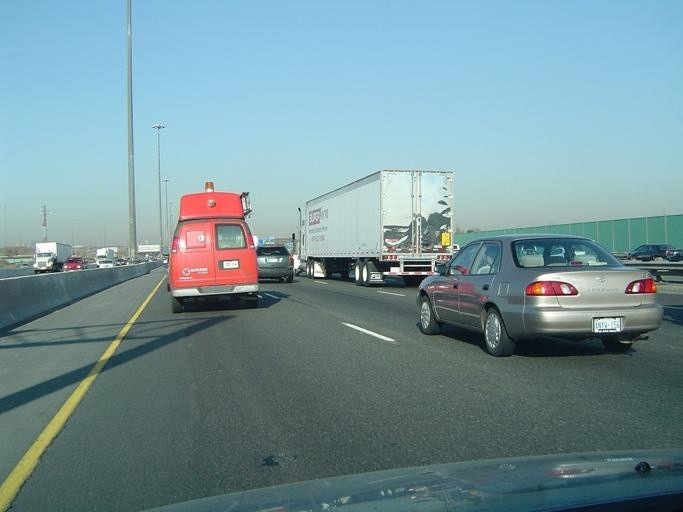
(256, 243), (294, 283)
(416, 233), (682, 357)
(95, 248), (127, 268)
(62, 256), (89, 272)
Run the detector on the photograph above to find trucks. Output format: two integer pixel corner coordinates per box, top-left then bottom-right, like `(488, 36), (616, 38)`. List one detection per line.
(32, 242), (72, 274)
(292, 169), (455, 286)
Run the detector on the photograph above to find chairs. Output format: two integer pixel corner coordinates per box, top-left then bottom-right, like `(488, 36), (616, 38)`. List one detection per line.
(519, 254), (598, 267)
(476, 251), (499, 274)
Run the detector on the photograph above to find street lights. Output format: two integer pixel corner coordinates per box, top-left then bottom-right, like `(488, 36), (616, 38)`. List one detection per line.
(152, 121), (167, 258)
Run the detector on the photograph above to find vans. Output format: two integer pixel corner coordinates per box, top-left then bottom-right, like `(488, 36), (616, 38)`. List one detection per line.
(161, 192), (259, 313)
